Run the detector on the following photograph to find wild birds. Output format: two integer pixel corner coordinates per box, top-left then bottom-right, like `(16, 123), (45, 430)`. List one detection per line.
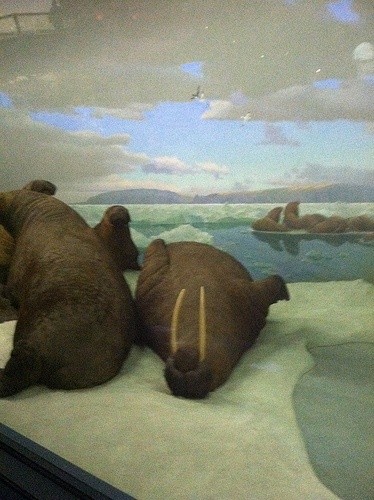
(190, 84), (203, 101)
(239, 112), (252, 127)
